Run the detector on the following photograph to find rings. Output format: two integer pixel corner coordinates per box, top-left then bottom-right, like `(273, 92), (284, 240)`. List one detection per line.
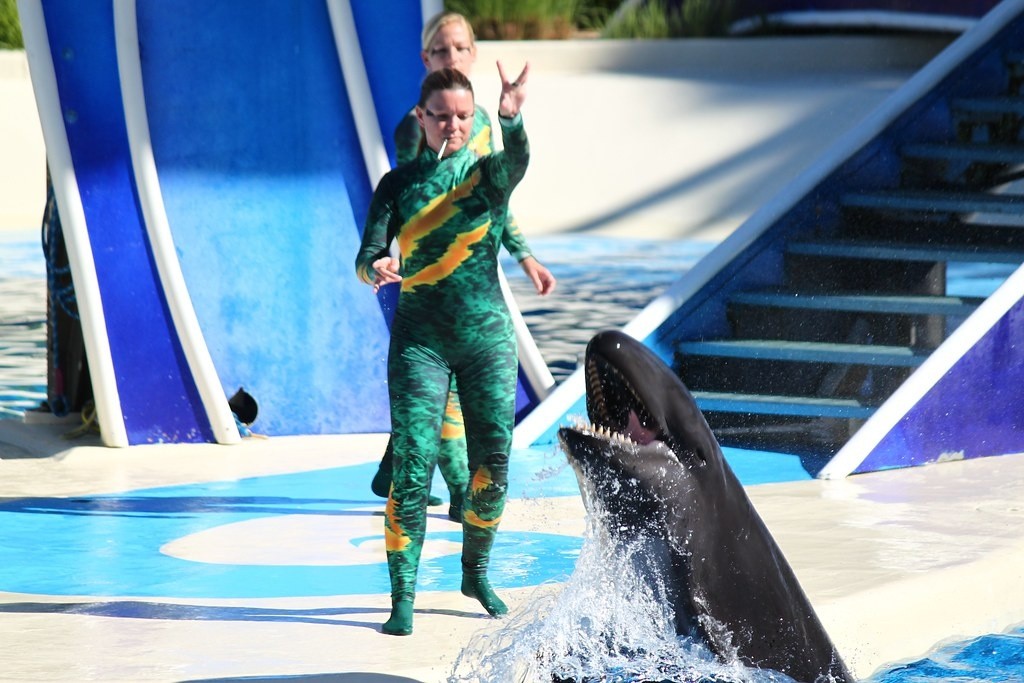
(512, 82), (519, 88)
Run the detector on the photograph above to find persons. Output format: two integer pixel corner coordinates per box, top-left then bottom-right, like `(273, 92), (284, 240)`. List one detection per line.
(355, 10), (557, 637)
